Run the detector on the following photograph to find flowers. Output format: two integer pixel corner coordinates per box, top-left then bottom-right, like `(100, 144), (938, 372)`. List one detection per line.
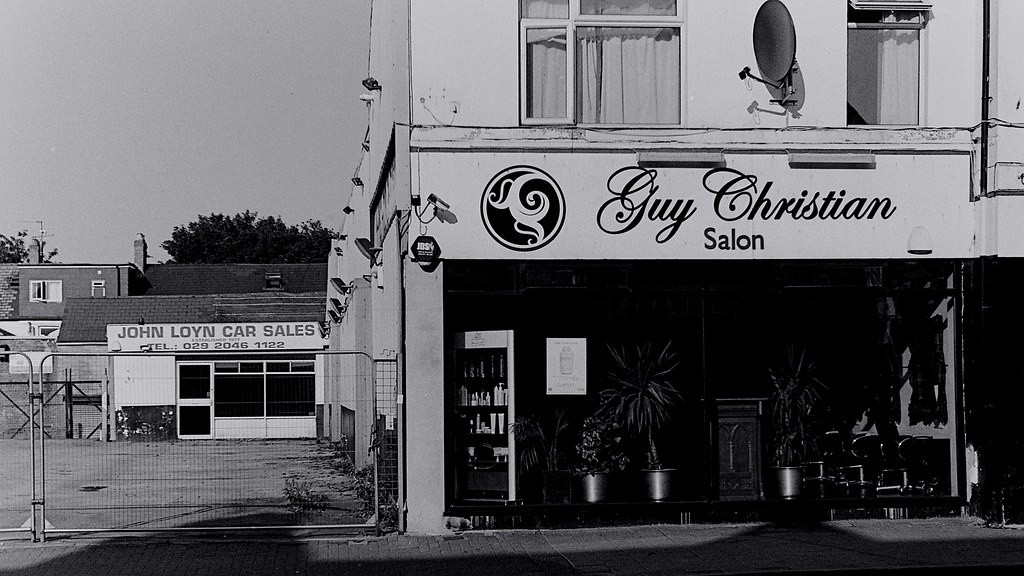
(574, 416), (630, 474)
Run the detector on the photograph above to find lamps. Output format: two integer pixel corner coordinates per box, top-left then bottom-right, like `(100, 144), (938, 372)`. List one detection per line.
(362, 77), (382, 91)
(328, 310), (342, 324)
(317, 328), (329, 338)
(600, 338), (682, 501)
(329, 277), (354, 295)
(329, 298), (348, 313)
(317, 320), (329, 334)
(355, 238), (383, 267)
(343, 206), (354, 214)
(338, 234), (347, 241)
(334, 247), (342, 253)
(351, 177), (364, 187)
(767, 347), (821, 496)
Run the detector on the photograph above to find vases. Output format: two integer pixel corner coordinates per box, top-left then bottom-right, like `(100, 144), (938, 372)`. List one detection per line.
(584, 471), (607, 502)
